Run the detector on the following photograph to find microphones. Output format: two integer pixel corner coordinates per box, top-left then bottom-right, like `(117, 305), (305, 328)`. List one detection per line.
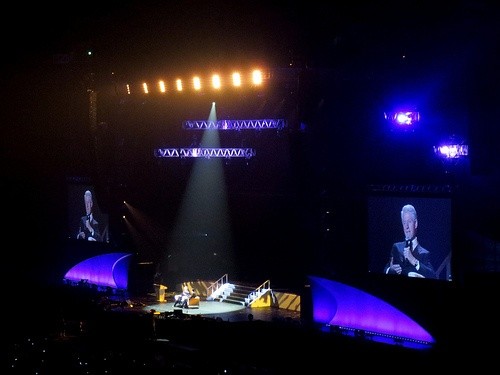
(405, 236), (412, 264)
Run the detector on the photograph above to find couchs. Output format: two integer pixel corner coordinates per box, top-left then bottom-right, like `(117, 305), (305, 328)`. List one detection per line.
(175, 295), (200, 308)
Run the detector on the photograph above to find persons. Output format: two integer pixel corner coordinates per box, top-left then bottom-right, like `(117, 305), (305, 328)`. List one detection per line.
(182, 289), (195, 309)
(76, 190), (102, 242)
(172, 286), (190, 308)
(384, 204), (439, 279)
